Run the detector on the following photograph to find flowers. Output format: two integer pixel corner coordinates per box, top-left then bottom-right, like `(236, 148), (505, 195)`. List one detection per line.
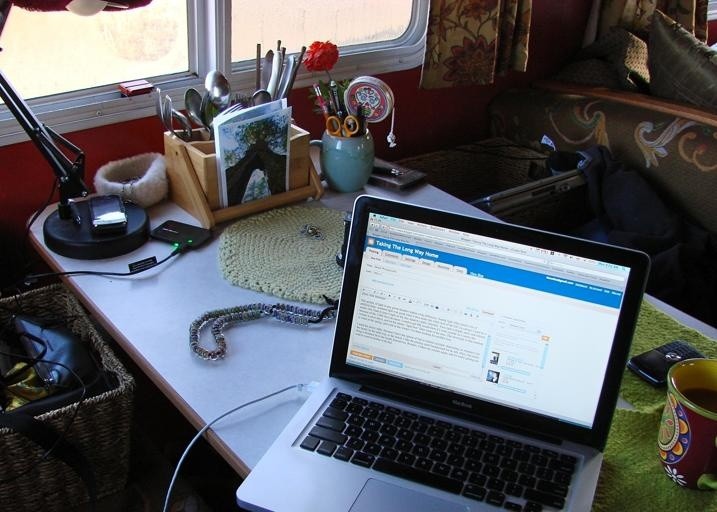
(303, 40), (350, 116)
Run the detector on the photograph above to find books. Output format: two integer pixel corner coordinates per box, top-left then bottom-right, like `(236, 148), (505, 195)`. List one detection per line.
(214, 98), (293, 208)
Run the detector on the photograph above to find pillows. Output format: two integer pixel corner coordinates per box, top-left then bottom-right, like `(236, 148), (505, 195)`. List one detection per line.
(558, 9), (717, 113)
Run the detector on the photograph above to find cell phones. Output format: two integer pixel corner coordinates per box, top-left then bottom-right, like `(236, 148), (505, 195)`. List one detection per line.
(89, 194), (128, 236)
(627, 340), (706, 388)
(150, 218), (214, 250)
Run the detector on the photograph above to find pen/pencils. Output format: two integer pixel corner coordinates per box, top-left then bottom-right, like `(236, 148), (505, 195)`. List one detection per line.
(371, 165), (404, 177)
(312, 80), (368, 137)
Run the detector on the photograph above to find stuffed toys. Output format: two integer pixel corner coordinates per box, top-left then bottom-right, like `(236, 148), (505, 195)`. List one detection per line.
(0, 0), (152, 43)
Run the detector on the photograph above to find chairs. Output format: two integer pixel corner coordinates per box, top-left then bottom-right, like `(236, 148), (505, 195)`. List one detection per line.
(473, 85), (717, 329)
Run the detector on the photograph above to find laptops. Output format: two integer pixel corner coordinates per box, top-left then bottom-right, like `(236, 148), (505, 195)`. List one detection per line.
(236, 193), (652, 511)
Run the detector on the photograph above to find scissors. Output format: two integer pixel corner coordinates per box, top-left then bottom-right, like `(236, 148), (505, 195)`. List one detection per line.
(325, 115), (360, 137)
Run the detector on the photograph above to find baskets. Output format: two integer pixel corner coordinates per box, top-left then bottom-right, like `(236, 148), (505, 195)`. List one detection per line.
(388, 135), (555, 206)
(0, 279), (137, 511)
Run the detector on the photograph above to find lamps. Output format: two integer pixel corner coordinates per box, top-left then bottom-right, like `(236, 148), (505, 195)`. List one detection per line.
(0, 0), (151, 261)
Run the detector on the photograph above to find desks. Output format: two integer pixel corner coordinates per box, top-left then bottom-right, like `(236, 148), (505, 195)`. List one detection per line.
(24, 175), (717, 512)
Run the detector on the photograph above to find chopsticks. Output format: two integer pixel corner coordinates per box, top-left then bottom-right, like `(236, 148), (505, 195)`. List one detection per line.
(249, 36), (308, 111)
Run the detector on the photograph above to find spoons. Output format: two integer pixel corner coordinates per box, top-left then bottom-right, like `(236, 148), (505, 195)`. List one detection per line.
(154, 69), (273, 141)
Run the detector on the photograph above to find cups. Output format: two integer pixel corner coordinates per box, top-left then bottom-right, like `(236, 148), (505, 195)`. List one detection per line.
(310, 129), (374, 193)
(658, 357), (717, 492)
